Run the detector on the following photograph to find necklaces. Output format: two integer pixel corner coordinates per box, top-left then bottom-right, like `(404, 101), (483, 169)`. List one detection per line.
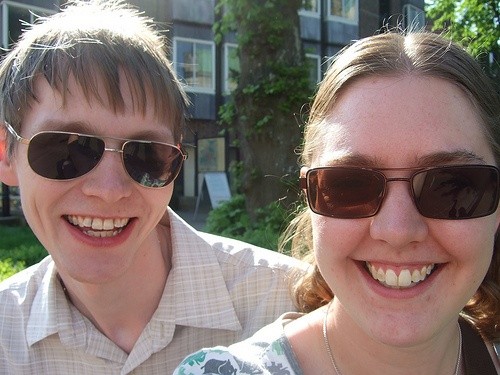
(323, 299), (462, 375)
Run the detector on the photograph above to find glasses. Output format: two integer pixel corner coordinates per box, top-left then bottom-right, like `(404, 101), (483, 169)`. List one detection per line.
(4, 121), (188, 190)
(296, 164), (500, 220)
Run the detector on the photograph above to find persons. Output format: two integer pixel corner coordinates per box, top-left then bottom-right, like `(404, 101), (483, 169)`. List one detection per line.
(174, 32), (500, 375)
(0, 1), (313, 375)
